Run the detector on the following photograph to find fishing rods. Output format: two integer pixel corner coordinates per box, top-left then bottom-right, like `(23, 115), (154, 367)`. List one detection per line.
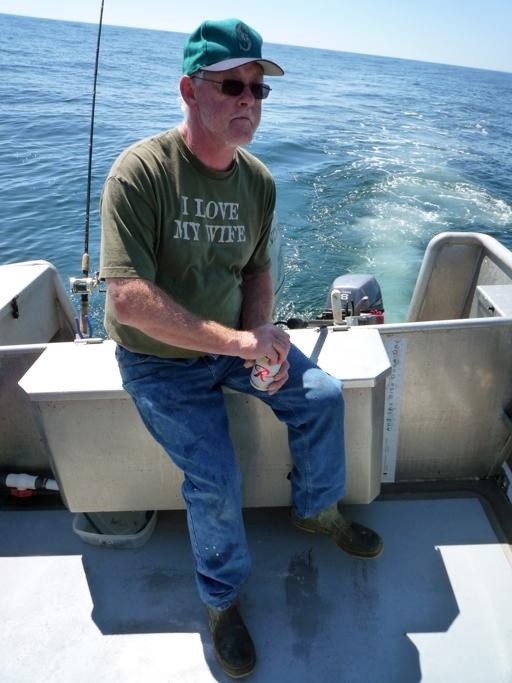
(70, 1), (106, 336)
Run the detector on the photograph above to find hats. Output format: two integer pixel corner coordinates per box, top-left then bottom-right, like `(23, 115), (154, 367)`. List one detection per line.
(183, 18), (284, 76)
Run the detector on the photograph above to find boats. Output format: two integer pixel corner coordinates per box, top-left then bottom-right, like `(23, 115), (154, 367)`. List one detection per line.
(0, 229), (512, 683)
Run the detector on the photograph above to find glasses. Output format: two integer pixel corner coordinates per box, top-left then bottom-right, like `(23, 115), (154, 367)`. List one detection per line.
(190, 76), (271, 99)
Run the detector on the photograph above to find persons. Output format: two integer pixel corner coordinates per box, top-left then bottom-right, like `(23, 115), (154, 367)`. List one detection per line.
(97, 16), (385, 679)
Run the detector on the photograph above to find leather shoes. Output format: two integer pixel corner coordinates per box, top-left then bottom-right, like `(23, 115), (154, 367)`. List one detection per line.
(291, 502), (384, 559)
(207, 605), (256, 678)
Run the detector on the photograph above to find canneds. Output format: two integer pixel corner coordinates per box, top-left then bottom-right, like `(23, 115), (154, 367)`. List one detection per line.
(249, 358), (282, 392)
